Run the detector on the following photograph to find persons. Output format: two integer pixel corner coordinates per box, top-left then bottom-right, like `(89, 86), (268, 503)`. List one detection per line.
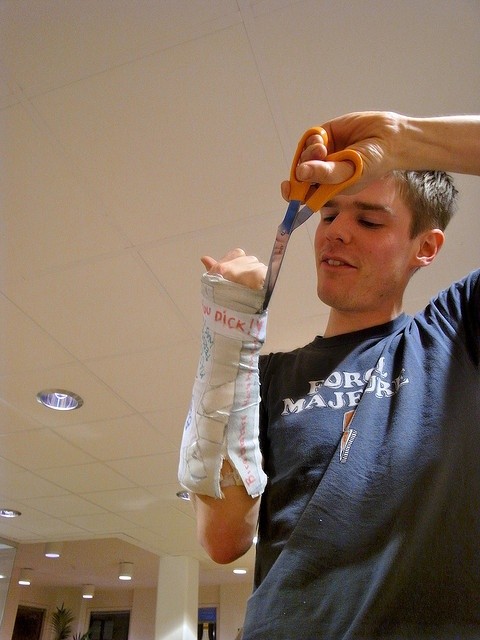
(176, 110), (479, 640)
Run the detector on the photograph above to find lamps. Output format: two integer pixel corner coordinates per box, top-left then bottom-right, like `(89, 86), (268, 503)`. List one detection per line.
(45, 541), (60, 557)
(118, 562), (134, 581)
(18, 568), (32, 586)
(81, 585), (94, 598)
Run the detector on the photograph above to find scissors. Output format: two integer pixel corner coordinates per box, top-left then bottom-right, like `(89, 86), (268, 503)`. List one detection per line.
(262, 126), (363, 310)
